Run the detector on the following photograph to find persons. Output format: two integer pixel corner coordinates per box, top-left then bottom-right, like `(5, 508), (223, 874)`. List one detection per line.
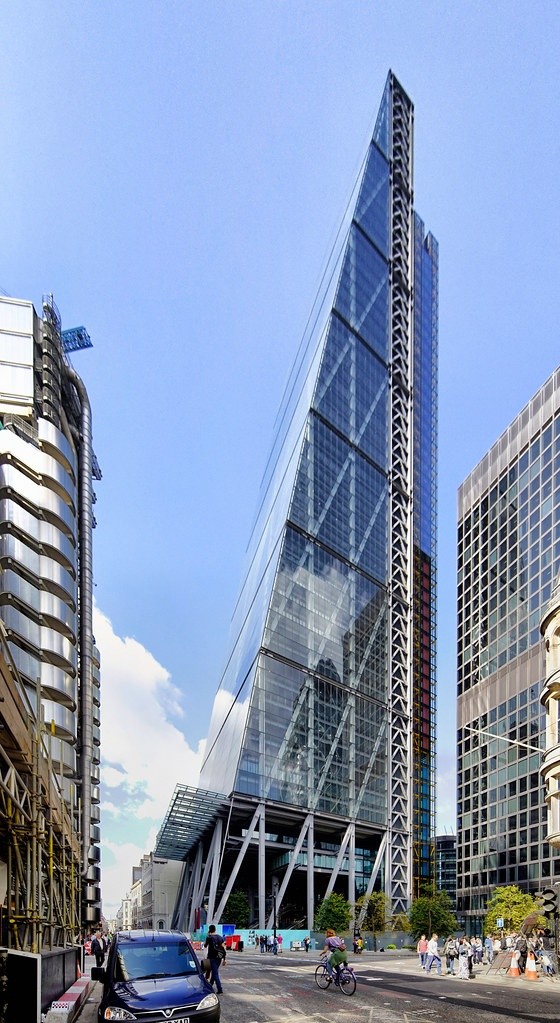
(417, 933), (482, 980)
(86, 934), (96, 941)
(101, 932), (112, 952)
(303, 936), (311, 952)
(255, 934), (283, 953)
(204, 925), (227, 994)
(91, 932), (107, 967)
(320, 929), (347, 982)
(485, 929), (555, 977)
(76, 934), (84, 945)
(352, 937), (363, 954)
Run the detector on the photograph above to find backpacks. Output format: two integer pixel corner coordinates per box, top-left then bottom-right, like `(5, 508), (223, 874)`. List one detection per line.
(213, 946), (226, 960)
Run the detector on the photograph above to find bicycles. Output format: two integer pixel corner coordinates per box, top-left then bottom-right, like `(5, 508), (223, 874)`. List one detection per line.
(314, 954), (358, 996)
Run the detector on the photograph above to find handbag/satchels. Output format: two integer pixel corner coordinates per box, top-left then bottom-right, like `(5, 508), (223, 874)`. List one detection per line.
(339, 943), (347, 951)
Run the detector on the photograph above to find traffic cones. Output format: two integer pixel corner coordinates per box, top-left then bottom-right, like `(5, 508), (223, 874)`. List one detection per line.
(508, 951), (521, 976)
(520, 952), (543, 983)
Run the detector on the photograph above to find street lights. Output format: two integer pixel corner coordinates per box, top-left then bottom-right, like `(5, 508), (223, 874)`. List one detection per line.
(269, 891), (278, 937)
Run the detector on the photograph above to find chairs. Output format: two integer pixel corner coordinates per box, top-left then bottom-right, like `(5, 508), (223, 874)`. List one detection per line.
(123, 952), (139, 971)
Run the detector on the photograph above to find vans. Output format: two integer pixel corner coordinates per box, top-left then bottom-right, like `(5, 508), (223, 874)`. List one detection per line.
(91, 928), (221, 1023)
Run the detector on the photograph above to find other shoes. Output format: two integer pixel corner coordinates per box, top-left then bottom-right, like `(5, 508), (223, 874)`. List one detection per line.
(217, 991), (223, 995)
(326, 977), (333, 983)
